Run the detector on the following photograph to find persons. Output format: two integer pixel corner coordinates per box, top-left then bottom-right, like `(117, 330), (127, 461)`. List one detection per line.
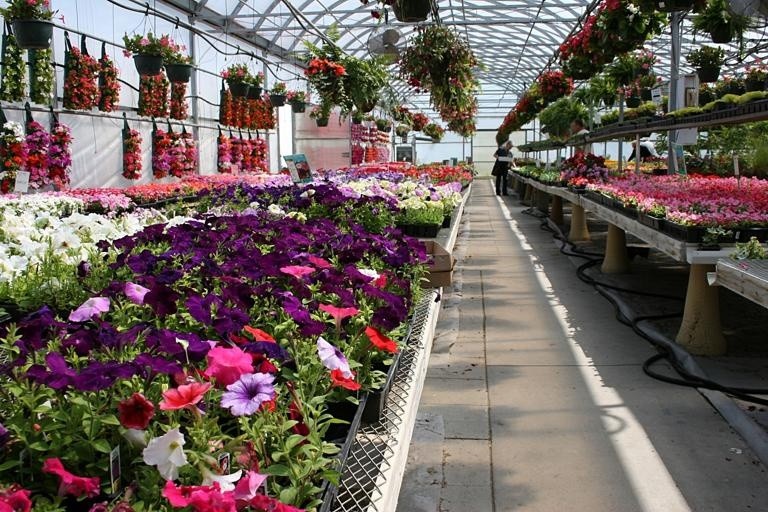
(570, 120), (594, 158)
(490, 139), (516, 197)
(627, 140), (653, 162)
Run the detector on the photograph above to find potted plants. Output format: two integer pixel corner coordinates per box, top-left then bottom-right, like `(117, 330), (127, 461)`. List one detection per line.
(0, 0), (57, 49)
(512, 166), (767, 245)
(610, 59), (659, 113)
(218, 63), (333, 130)
(686, 47), (729, 86)
(120, 27), (199, 83)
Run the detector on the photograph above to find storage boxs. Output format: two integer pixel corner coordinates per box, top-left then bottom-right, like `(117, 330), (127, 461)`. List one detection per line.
(415, 240), (457, 292)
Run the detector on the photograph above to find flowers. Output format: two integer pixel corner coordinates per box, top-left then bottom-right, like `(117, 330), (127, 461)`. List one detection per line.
(215, 135), (272, 176)
(119, 125), (202, 184)
(136, 77), (192, 123)
(1, 180), (464, 512)
(63, 49), (121, 115)
(0, 44), (78, 197)
(217, 89), (276, 130)
